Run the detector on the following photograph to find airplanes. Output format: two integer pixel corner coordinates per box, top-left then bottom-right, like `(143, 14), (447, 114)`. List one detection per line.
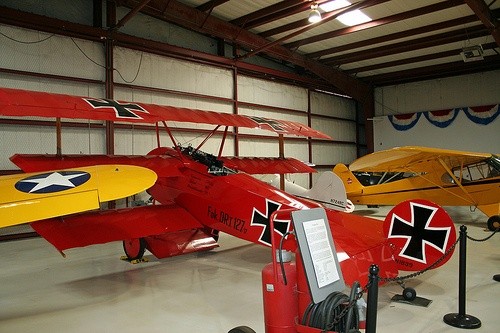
(0, 87), (500, 333)
